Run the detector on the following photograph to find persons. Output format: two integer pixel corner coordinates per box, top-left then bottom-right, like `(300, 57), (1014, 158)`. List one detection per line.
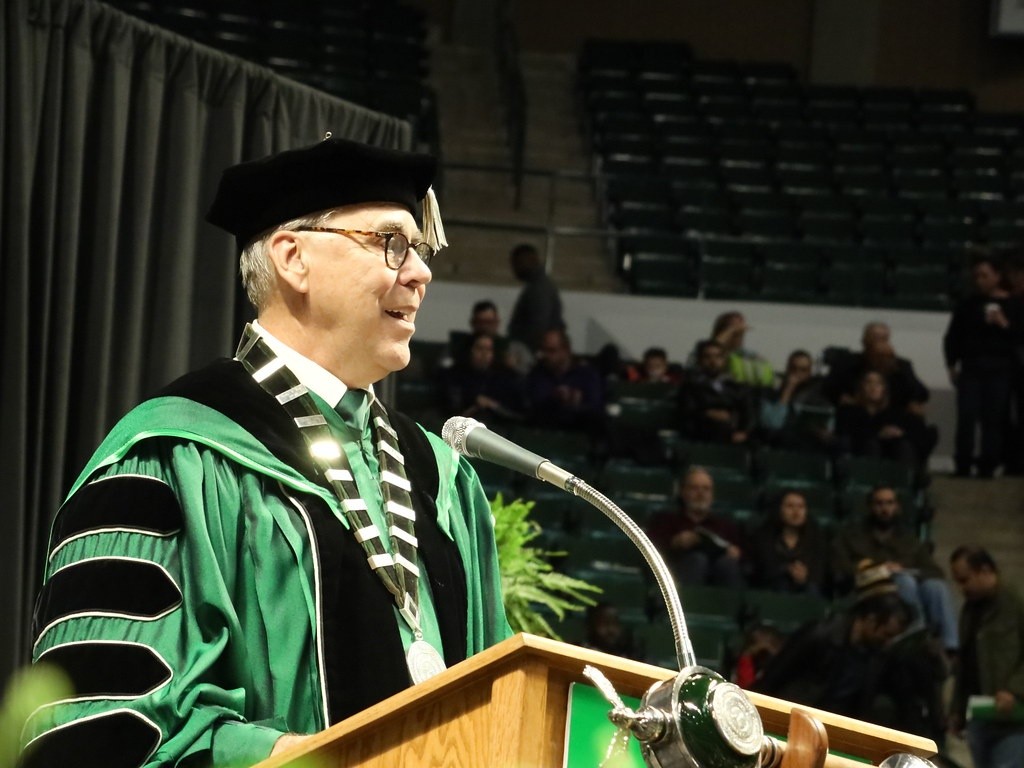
(1, 130), (516, 768)
(443, 246), (1024, 768)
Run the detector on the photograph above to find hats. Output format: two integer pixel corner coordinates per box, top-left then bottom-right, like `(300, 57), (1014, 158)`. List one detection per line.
(202, 131), (449, 257)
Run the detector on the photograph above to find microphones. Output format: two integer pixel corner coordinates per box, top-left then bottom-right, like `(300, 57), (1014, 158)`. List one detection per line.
(443, 416), (697, 671)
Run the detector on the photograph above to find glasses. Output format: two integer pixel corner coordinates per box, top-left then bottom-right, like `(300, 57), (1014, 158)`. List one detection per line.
(290, 225), (436, 271)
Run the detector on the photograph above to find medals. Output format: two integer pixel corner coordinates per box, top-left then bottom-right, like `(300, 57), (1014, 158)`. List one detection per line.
(407, 641), (447, 685)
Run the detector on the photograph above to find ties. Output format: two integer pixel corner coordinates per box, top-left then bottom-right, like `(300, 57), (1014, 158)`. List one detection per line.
(335, 388), (380, 492)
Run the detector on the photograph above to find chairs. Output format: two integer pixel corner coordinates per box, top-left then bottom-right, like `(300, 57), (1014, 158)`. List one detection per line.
(573, 39), (1023, 315)
(116, 0), (440, 174)
(400, 334), (928, 671)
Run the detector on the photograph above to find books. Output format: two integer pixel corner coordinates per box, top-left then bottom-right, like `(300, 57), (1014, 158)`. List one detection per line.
(966, 694), (1022, 723)
